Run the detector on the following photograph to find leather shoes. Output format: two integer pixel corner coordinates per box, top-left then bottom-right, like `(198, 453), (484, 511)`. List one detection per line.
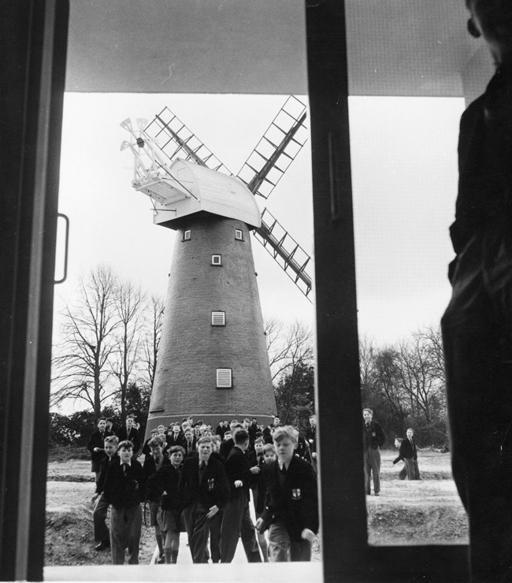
(95, 542), (105, 551)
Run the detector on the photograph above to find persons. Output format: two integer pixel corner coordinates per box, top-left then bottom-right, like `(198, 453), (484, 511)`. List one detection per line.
(393, 427), (421, 481)
(439, 0), (512, 583)
(86, 412), (318, 566)
(362, 407), (386, 496)
